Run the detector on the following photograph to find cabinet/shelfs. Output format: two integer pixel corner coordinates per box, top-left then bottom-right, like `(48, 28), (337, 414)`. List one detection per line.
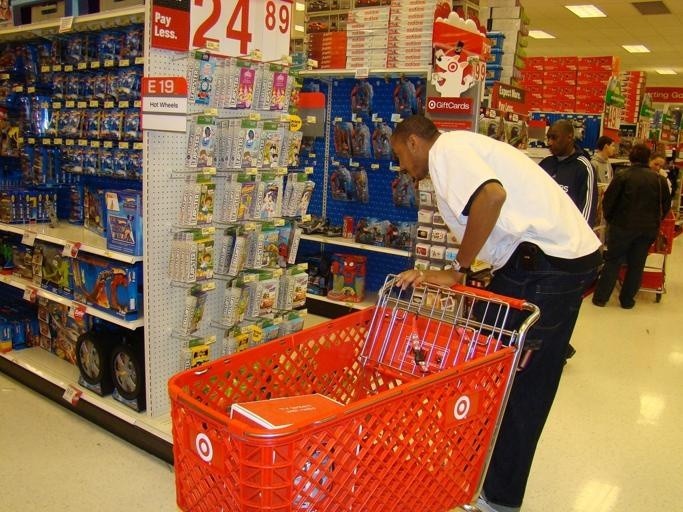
(296, 66), (423, 306)
(0, 0), (146, 420)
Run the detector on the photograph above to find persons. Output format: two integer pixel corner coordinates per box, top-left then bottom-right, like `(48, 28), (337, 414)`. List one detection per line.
(390, 113), (604, 512)
(592, 135), (680, 309)
(538, 120), (598, 358)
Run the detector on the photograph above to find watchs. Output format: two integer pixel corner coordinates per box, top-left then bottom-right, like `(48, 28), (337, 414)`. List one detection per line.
(450, 259), (472, 275)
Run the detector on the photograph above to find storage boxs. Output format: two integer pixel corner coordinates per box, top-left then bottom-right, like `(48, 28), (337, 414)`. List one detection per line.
(389, 1), (452, 68)
(230, 392), (362, 511)
(345, 6), (390, 70)
(302, 32), (346, 68)
(479, 3), (529, 90)
(524, 55), (648, 131)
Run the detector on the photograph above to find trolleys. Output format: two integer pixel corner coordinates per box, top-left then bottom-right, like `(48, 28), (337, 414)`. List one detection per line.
(618, 208), (678, 304)
(162, 268), (537, 511)
